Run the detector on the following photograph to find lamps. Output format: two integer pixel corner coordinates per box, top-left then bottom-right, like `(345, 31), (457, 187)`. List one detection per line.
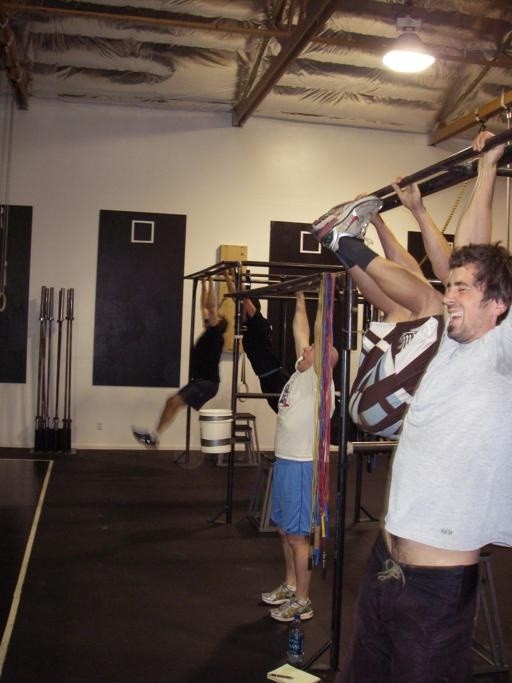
(382, 0), (436, 73)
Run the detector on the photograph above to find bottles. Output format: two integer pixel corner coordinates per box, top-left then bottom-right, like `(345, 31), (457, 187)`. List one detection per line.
(313, 547), (320, 567)
(287, 612), (303, 664)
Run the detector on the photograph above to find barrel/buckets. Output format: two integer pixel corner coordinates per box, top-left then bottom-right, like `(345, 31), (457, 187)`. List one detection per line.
(198, 408), (234, 454)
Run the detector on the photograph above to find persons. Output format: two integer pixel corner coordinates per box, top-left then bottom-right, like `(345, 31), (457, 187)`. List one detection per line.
(220, 262), (291, 412)
(309, 129), (505, 440)
(340, 128), (512, 681)
(131, 273), (228, 450)
(261, 271), (340, 623)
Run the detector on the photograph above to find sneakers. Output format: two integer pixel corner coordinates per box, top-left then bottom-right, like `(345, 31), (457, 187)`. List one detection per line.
(311, 198), (354, 233)
(315, 191), (385, 254)
(267, 594), (316, 623)
(133, 430), (160, 449)
(261, 581), (297, 605)
